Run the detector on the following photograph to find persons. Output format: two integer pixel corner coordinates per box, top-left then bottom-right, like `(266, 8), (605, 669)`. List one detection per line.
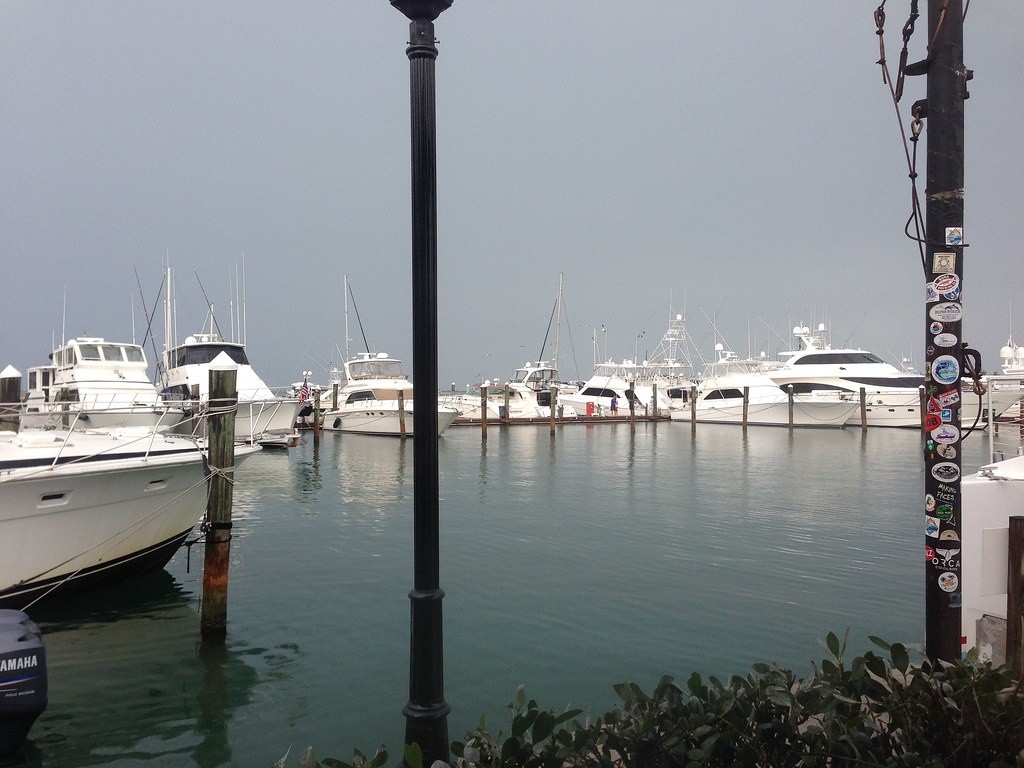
(611, 396), (618, 416)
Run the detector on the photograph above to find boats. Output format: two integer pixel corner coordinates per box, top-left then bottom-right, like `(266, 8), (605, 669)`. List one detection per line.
(0, 399), (285, 603)
(282, 273), (461, 439)
(441, 271), (579, 422)
(15, 285), (184, 435)
(155, 255), (316, 448)
(558, 305), (1024, 430)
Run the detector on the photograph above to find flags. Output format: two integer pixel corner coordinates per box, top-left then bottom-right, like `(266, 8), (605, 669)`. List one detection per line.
(300, 379), (308, 406)
